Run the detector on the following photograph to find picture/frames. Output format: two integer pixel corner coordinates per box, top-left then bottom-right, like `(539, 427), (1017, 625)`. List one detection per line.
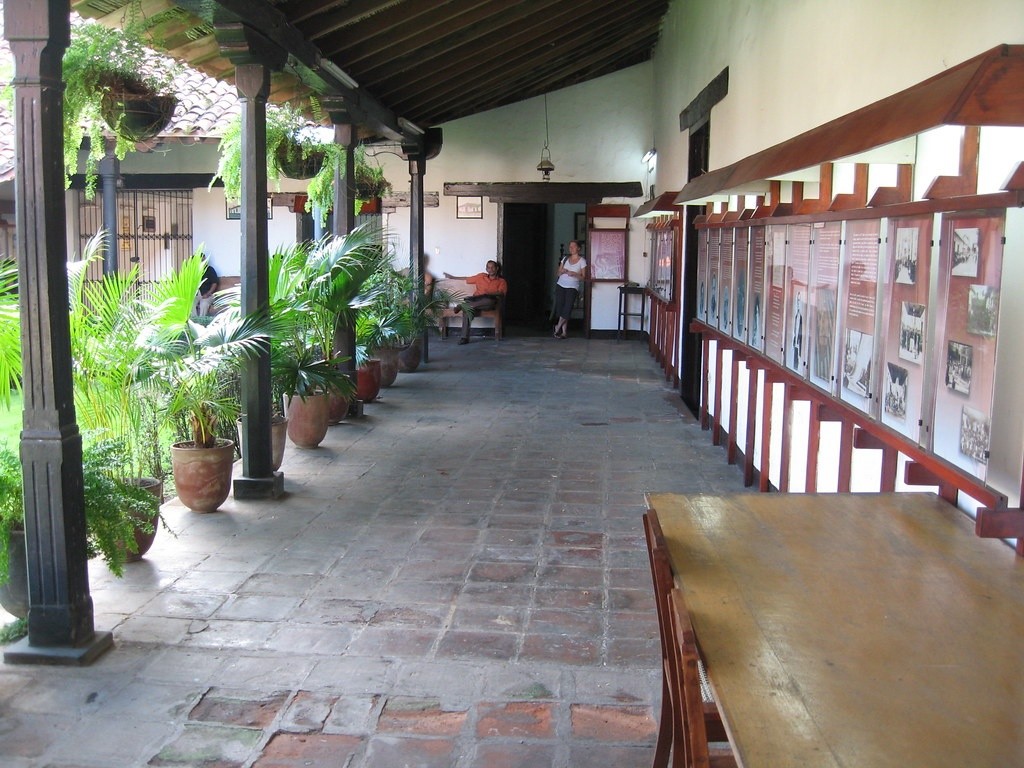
(226, 197), (272, 220)
(456, 196), (483, 220)
(574, 212), (586, 242)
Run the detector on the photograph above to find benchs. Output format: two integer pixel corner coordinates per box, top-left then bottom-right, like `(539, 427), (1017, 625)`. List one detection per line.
(422, 276), (504, 342)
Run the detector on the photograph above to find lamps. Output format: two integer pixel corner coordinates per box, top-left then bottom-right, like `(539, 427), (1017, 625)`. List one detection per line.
(537, 145), (554, 183)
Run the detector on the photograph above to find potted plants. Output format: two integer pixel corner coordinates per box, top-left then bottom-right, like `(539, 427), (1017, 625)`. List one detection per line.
(305, 144), (388, 227)
(0, 213), (475, 627)
(207, 100), (356, 205)
(55, 0), (214, 201)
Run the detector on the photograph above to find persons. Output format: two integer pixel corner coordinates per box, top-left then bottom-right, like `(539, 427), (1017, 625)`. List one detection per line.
(552, 240), (588, 339)
(443, 260), (508, 345)
(191, 253), (218, 317)
(388, 266), (433, 309)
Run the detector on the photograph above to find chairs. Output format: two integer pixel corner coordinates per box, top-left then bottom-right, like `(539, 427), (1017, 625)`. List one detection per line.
(668, 586), (738, 767)
(643, 508), (729, 767)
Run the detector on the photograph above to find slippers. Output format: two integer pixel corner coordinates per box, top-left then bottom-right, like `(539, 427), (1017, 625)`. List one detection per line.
(557, 335), (568, 339)
(553, 325), (557, 339)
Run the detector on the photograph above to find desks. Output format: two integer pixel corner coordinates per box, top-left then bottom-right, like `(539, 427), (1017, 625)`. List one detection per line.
(618, 286), (645, 342)
(644, 488), (1023, 768)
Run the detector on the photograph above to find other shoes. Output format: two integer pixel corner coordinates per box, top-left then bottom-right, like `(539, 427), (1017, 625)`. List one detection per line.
(454, 304), (462, 314)
(458, 338), (469, 345)
